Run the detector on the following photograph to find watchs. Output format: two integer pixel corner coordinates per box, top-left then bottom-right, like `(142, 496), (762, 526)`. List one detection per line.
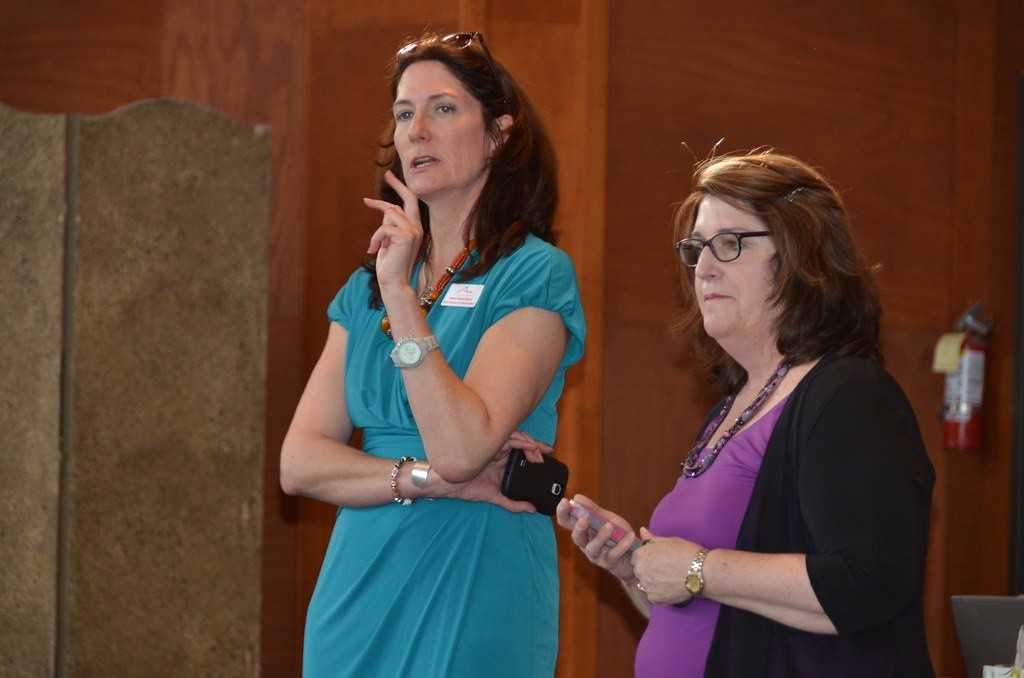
(389, 334), (440, 369)
(683, 547), (711, 599)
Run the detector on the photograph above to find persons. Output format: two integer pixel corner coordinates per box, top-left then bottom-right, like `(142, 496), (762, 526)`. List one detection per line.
(553, 149), (935, 677)
(278, 29), (590, 678)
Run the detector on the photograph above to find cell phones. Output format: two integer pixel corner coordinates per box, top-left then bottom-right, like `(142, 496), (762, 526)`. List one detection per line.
(500, 448), (569, 516)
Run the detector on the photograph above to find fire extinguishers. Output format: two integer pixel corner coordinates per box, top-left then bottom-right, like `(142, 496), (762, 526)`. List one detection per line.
(941, 301), (990, 450)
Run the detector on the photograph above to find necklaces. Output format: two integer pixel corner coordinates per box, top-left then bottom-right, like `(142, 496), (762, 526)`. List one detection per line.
(380, 232), (479, 340)
(680, 356), (798, 479)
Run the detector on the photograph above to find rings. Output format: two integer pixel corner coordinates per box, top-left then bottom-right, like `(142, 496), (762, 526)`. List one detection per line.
(637, 583), (646, 595)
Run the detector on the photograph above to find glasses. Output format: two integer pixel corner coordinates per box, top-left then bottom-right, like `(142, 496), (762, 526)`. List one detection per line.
(397, 30), (508, 109)
(680, 230), (775, 267)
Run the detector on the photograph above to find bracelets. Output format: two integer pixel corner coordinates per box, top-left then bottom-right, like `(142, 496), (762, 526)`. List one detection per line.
(411, 459), (435, 501)
(390, 456), (418, 506)
